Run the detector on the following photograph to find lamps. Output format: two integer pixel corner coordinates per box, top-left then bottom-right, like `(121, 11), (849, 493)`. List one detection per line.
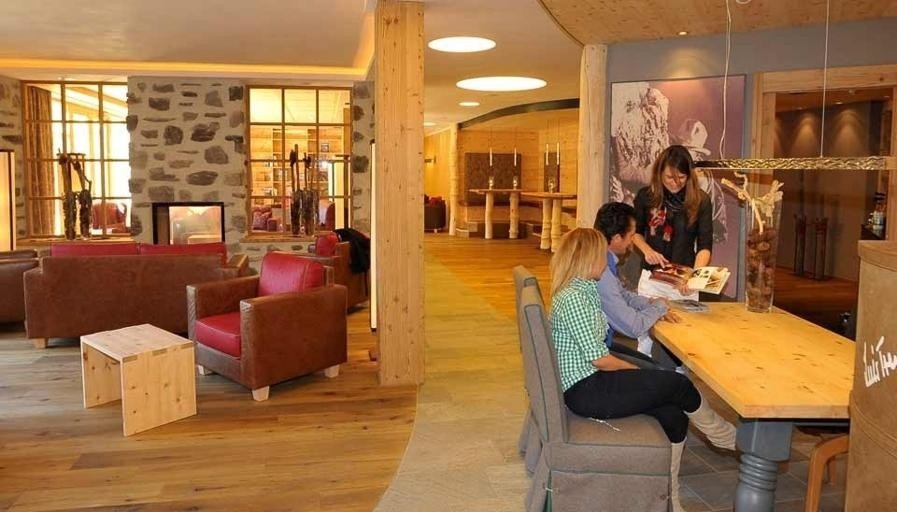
(486, 119), (571, 167)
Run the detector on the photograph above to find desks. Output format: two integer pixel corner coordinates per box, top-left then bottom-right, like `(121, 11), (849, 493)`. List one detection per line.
(468, 189), (526, 253)
(521, 190), (577, 254)
(651, 302), (857, 512)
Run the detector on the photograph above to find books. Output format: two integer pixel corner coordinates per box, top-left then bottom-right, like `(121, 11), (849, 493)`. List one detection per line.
(647, 258), (731, 296)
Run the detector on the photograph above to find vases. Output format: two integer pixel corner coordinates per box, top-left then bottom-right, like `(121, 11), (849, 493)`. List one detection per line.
(744, 229), (783, 313)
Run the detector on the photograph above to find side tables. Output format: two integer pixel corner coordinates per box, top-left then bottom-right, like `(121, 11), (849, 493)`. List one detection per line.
(80, 323), (197, 438)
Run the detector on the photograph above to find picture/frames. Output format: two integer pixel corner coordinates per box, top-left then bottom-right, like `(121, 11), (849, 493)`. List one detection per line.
(609, 73), (747, 300)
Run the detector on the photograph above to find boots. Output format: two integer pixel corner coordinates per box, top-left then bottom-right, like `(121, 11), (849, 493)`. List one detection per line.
(671, 435), (688, 512)
(681, 387), (737, 451)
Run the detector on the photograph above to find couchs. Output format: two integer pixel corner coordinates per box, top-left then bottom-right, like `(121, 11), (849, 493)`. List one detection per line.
(23, 242), (248, 349)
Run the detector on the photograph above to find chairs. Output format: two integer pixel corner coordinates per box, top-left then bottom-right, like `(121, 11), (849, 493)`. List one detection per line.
(185, 252), (350, 401)
(622, 253), (641, 288)
(249, 203), (288, 235)
(518, 285), (673, 512)
(316, 200), (336, 231)
(91, 202), (127, 231)
(424, 199), (446, 233)
(296, 231), (370, 315)
(513, 265), (549, 476)
(796, 423), (848, 512)
(0, 249), (39, 328)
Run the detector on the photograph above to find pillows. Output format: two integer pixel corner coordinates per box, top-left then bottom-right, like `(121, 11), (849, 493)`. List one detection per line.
(316, 232), (340, 257)
(252, 203), (273, 219)
(49, 242), (139, 255)
(138, 243), (225, 254)
(253, 212), (270, 230)
(267, 217), (277, 231)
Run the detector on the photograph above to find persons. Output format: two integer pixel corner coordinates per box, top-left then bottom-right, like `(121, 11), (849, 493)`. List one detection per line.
(630, 143), (713, 375)
(587, 201), (689, 378)
(545, 226), (738, 511)
